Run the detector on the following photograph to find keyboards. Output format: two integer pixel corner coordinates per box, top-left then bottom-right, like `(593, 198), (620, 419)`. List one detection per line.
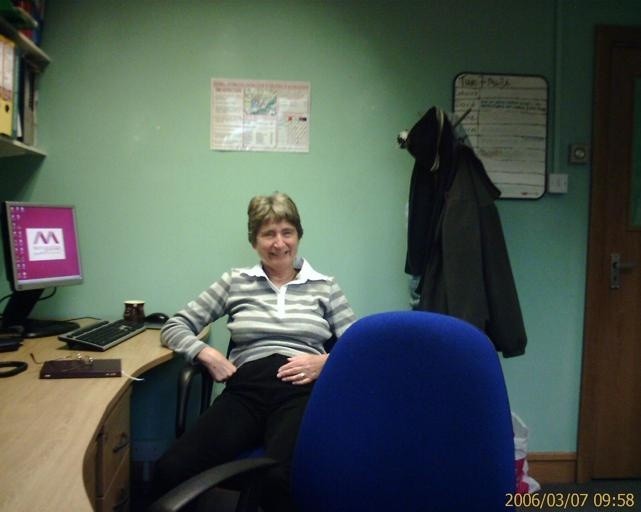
(58, 318), (146, 352)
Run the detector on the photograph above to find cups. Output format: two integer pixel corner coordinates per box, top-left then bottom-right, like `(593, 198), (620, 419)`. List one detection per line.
(123, 299), (145, 325)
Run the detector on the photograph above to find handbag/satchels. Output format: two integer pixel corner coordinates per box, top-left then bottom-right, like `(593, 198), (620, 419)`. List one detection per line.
(510, 411), (541, 499)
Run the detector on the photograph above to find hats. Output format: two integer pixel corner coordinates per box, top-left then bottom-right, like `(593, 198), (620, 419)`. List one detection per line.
(399, 105), (450, 174)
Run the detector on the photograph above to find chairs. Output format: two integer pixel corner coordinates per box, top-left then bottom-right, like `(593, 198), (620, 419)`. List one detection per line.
(151, 311), (514, 511)
(172, 335), (336, 497)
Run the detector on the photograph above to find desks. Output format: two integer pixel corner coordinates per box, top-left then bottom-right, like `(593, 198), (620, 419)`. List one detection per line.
(0, 319), (211, 512)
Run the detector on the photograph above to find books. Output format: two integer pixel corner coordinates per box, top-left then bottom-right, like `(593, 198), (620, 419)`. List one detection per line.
(0, 35), (41, 146)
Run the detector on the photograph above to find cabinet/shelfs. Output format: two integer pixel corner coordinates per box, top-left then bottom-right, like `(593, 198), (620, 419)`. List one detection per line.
(0, 0), (53, 158)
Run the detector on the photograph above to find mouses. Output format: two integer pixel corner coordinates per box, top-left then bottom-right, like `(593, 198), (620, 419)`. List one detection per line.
(145, 313), (169, 323)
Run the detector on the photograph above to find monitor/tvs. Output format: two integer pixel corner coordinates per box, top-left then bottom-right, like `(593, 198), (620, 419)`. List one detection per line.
(2, 201), (84, 338)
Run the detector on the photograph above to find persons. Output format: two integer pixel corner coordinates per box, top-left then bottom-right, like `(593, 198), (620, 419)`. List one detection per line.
(157, 191), (358, 510)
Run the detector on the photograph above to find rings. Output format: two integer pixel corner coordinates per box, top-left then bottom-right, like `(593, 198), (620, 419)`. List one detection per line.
(301, 372), (306, 378)
